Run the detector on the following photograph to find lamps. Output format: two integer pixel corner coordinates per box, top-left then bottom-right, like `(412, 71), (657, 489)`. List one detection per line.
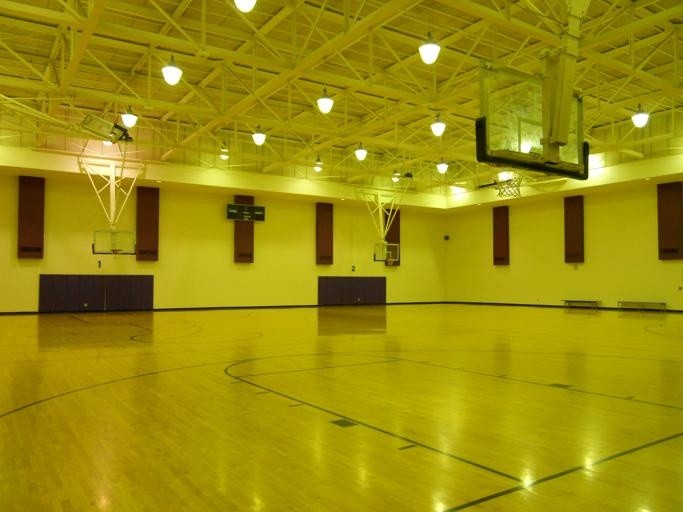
(251, 127), (266, 146)
(317, 86), (333, 116)
(119, 104), (139, 128)
(160, 55), (183, 87)
(631, 102), (649, 130)
(353, 142), (369, 162)
(417, 28), (449, 176)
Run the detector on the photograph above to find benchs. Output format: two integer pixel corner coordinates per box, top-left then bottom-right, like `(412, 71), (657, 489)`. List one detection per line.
(560, 297), (666, 314)
